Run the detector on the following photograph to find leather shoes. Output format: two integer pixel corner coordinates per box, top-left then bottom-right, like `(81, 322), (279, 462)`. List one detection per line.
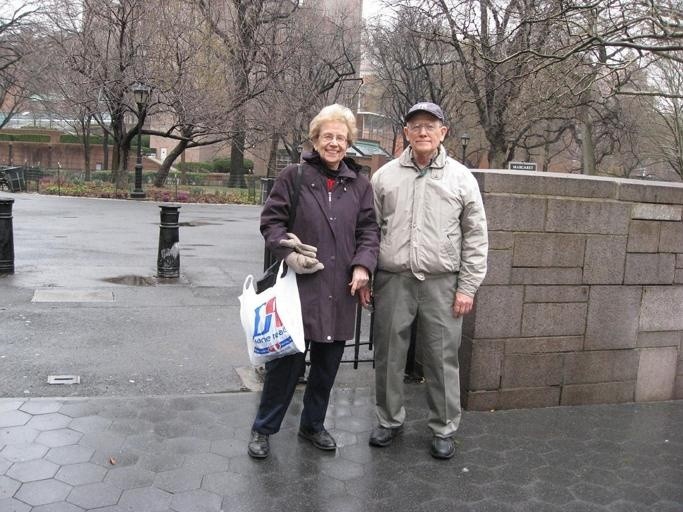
(248, 429), (270, 458)
(299, 425), (336, 450)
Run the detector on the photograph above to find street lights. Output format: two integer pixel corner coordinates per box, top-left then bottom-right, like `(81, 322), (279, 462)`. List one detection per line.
(460, 131), (470, 166)
(130, 81), (149, 199)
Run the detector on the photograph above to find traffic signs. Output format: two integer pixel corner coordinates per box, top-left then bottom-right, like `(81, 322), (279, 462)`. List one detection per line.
(509, 162), (537, 172)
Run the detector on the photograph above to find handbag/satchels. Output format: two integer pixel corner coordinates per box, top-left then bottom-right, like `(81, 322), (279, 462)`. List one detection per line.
(256, 261), (280, 295)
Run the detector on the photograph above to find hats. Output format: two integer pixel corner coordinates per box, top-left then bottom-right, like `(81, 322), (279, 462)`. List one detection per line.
(404, 102), (444, 124)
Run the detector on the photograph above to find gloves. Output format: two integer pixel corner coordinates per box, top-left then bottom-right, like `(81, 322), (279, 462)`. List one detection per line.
(285, 251), (325, 274)
(280, 232), (318, 257)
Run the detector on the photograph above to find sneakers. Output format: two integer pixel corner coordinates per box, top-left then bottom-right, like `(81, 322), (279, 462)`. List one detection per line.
(430, 436), (455, 458)
(369, 423), (404, 446)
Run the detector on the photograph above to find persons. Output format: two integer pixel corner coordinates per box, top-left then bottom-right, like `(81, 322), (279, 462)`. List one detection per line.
(359, 103), (489, 458)
(249, 103), (379, 458)
